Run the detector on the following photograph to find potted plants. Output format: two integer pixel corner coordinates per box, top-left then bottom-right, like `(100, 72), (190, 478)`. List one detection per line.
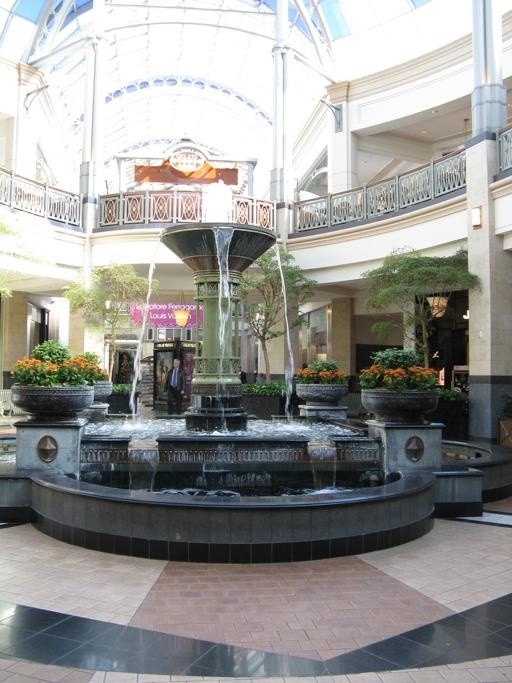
(424, 388), (467, 424)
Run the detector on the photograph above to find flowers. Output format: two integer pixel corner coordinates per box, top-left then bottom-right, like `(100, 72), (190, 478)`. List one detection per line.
(359, 347), (439, 389)
(295, 359), (344, 383)
(10, 357), (110, 387)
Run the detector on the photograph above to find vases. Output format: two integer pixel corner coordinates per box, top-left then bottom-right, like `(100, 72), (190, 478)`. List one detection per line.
(94, 380), (114, 401)
(294, 382), (350, 403)
(360, 388), (445, 416)
(9, 384), (95, 413)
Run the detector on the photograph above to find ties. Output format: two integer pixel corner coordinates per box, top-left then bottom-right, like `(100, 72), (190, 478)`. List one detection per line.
(172, 369), (177, 388)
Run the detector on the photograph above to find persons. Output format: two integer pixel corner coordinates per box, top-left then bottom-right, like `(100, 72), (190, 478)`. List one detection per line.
(163, 358), (186, 415)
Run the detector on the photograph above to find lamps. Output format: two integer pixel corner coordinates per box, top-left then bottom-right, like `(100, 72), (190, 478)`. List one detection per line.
(172, 290), (191, 327)
